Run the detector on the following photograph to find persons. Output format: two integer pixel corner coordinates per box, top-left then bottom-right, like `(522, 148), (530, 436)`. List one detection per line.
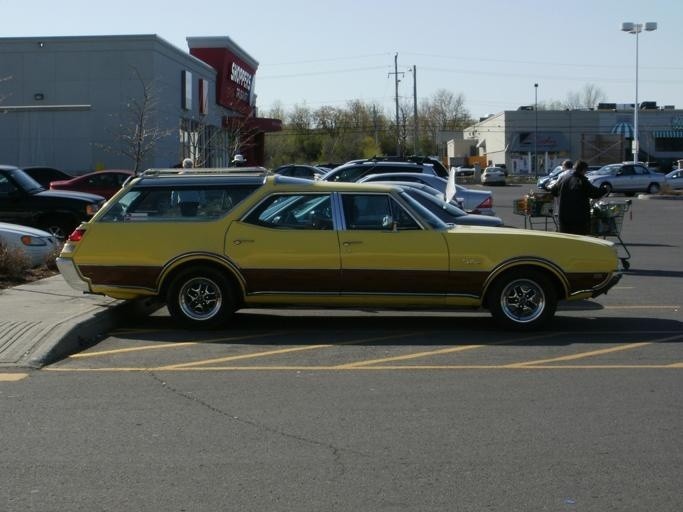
(222, 153), (251, 205)
(551, 159), (574, 231)
(171, 158), (208, 218)
(550, 159), (609, 236)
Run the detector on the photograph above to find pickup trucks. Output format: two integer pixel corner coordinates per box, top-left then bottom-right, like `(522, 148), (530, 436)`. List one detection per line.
(56, 167), (624, 331)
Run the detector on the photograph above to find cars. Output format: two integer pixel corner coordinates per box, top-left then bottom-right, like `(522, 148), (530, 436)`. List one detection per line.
(3, 167), (78, 190)
(262, 186), (503, 226)
(583, 163), (665, 196)
(49, 170), (138, 201)
(536, 165), (601, 190)
(664, 168), (682, 189)
(274, 155), (506, 184)
(355, 173), (494, 216)
(0, 165), (106, 239)
(0, 221), (61, 272)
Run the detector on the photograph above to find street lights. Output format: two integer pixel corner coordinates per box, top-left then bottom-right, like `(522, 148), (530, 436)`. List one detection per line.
(533, 82), (539, 171)
(619, 21), (657, 164)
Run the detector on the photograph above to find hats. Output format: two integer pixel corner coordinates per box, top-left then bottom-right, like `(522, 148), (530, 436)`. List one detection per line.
(231, 154), (247, 163)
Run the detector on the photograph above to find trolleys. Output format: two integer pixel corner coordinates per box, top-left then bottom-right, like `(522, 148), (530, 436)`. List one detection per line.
(589, 199), (632, 270)
(511, 189), (558, 233)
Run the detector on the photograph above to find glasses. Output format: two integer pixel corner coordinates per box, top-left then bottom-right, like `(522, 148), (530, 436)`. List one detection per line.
(236, 163), (244, 167)
